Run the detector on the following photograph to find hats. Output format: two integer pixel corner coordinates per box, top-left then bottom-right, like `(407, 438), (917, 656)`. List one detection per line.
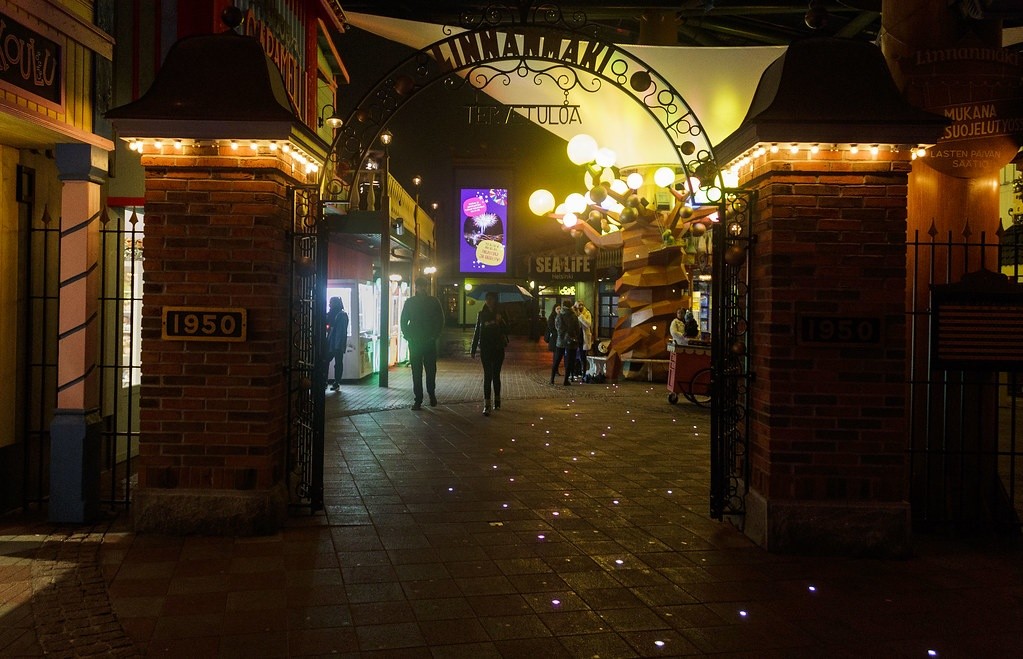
(684, 310), (693, 319)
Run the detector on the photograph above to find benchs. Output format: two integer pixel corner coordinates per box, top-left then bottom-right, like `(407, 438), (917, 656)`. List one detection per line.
(586, 355), (670, 382)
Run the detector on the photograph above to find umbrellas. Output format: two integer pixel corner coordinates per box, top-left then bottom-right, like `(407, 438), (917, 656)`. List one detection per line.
(467, 283), (535, 309)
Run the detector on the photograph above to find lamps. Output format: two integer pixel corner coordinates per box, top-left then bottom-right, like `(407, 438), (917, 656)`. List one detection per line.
(319, 104), (344, 128)
(529, 133), (720, 254)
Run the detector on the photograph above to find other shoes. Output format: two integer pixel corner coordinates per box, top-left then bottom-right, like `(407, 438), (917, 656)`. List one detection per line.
(482, 399), (492, 414)
(493, 394), (500, 409)
(411, 401), (421, 411)
(429, 396), (437, 406)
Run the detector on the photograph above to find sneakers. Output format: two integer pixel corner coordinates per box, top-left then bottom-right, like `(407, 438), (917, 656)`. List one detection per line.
(325, 382), (329, 391)
(330, 381), (340, 390)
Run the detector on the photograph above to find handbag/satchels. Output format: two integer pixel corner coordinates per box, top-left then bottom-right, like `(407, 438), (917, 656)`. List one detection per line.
(564, 334), (579, 352)
(543, 326), (550, 343)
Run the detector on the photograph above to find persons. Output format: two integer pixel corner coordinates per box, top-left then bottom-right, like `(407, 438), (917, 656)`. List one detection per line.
(325, 297), (349, 390)
(670, 308), (698, 345)
(545, 301), (593, 386)
(470, 293), (511, 416)
(401, 277), (445, 410)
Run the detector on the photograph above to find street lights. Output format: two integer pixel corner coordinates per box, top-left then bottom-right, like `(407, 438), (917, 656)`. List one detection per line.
(409, 172), (428, 294)
(378, 127), (396, 390)
(430, 200), (447, 331)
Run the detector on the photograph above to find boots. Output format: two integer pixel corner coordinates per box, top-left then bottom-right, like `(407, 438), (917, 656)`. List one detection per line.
(550, 367), (556, 384)
(563, 369), (571, 385)
